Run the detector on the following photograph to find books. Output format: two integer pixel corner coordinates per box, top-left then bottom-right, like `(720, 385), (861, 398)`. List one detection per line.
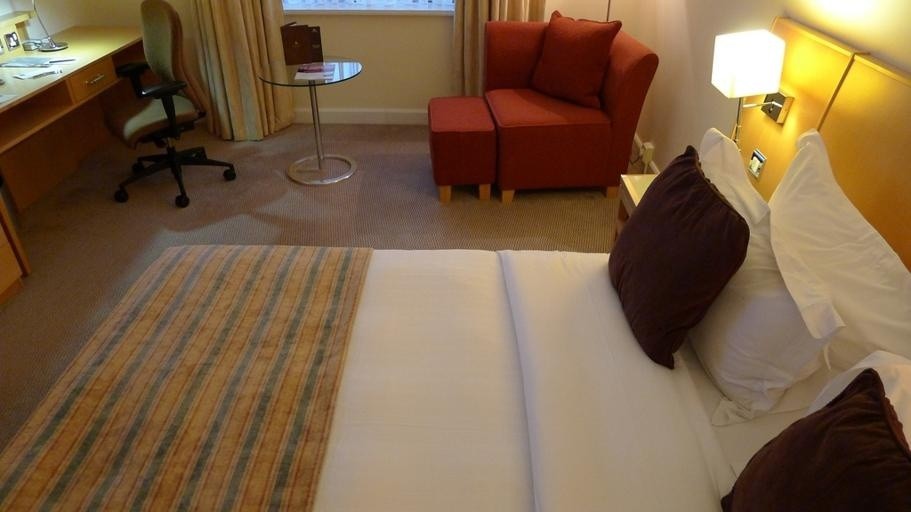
(279, 22), (323, 65)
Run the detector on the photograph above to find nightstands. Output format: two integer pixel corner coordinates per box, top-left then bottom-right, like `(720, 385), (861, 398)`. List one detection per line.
(611, 174), (659, 246)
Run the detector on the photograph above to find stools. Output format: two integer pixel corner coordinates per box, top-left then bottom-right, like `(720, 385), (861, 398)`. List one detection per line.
(427, 96), (496, 202)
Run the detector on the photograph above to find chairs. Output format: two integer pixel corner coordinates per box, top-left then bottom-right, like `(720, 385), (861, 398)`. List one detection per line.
(105, 0), (236, 208)
(483, 21), (658, 202)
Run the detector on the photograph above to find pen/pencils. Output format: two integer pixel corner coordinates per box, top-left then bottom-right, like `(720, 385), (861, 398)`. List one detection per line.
(49, 58), (76, 63)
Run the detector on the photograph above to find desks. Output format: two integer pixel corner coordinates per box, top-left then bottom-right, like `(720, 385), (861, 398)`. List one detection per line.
(257, 55), (362, 185)
(1, 11), (143, 276)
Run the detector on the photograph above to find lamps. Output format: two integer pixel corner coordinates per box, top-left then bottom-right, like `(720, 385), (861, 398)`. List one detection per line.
(710, 29), (794, 144)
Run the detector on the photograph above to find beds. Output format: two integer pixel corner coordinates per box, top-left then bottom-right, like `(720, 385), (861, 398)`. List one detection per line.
(2, 16), (911, 512)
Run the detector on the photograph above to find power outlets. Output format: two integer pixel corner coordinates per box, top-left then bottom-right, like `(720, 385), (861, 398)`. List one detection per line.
(639, 143), (653, 164)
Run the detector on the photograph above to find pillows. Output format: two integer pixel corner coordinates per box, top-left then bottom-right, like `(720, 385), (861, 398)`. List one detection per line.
(721, 366), (911, 512)
(688, 127), (845, 428)
(609, 146), (750, 372)
(531, 10), (621, 109)
(802, 351), (911, 450)
(768, 128), (911, 413)
